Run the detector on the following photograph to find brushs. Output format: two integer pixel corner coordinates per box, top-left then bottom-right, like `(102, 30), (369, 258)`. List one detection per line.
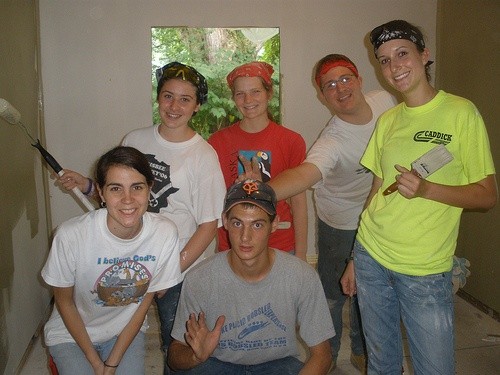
(382, 144), (454, 196)
(0, 99), (97, 212)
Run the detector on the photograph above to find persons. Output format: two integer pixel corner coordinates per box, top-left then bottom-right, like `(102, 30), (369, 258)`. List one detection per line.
(167, 179), (335, 375)
(40, 144), (183, 375)
(336, 19), (499, 375)
(207, 61), (308, 264)
(55, 60), (228, 355)
(231, 53), (405, 375)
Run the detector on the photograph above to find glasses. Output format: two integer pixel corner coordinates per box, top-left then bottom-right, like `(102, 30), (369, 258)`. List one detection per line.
(321, 75), (356, 90)
(225, 186), (276, 210)
(369, 18), (422, 45)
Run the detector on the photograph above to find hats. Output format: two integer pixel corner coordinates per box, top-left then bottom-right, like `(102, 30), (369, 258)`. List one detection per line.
(223, 178), (277, 216)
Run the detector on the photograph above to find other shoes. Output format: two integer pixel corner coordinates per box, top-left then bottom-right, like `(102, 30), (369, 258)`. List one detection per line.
(350, 351), (367, 374)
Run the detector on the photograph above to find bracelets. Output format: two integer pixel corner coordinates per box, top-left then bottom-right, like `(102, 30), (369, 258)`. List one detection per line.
(82, 177), (92, 195)
(104, 361), (119, 367)
(345, 256), (354, 264)
